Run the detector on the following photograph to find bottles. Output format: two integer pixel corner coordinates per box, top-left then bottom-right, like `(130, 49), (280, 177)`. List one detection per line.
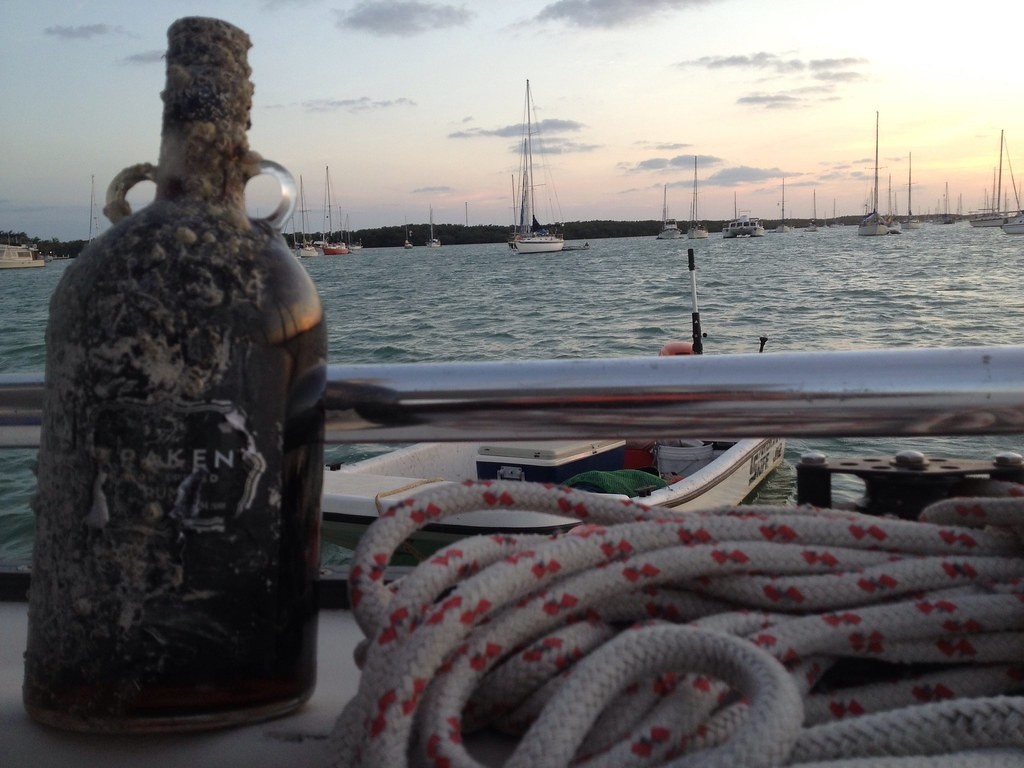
(23, 18), (332, 732)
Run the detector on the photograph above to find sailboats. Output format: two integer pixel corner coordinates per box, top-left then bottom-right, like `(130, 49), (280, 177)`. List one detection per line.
(289, 78), (1024, 258)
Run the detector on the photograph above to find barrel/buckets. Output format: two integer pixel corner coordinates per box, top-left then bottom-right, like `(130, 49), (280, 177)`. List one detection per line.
(658, 442), (713, 483)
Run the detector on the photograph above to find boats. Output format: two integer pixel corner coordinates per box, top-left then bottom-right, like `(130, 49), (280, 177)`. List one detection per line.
(0, 242), (46, 269)
(312, 244), (789, 561)
